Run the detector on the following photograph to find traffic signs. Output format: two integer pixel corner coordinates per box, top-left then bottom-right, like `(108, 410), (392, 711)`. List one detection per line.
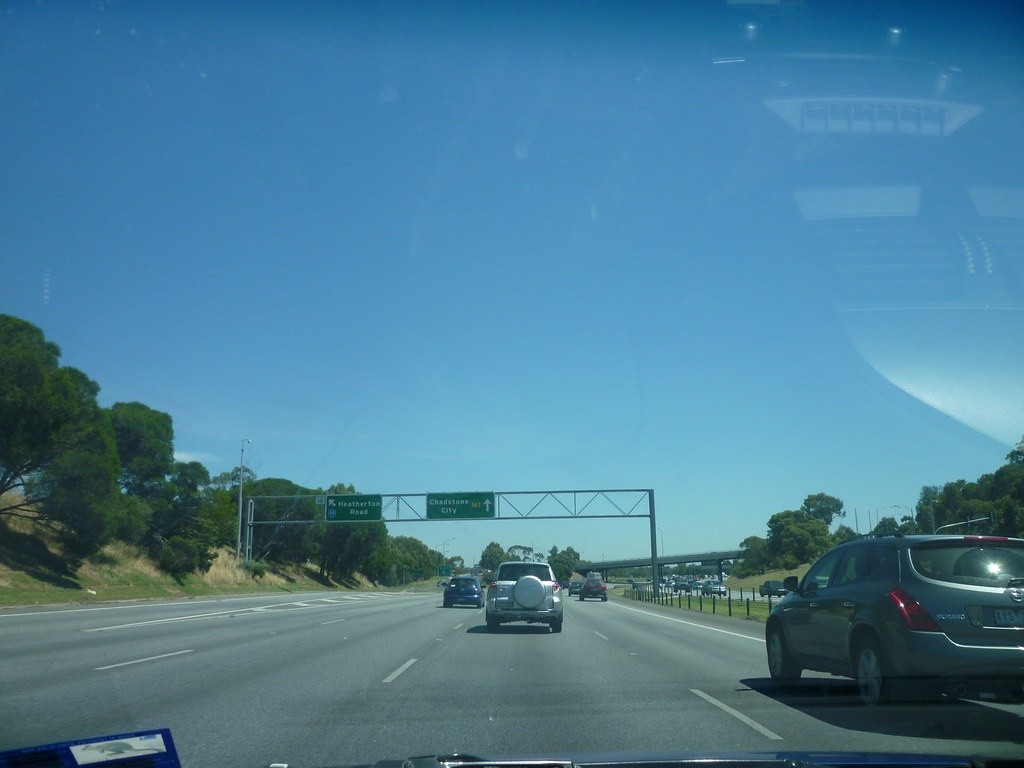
(326, 494), (383, 522)
(425, 491), (495, 519)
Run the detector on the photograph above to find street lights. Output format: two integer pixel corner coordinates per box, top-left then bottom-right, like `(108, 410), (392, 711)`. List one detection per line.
(234, 438), (254, 569)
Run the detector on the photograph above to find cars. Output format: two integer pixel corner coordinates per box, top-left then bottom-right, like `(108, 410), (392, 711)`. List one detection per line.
(441, 574), (486, 610)
(764, 533), (1024, 716)
(692, 581), (704, 591)
(563, 581), (572, 588)
(568, 581), (585, 596)
(648, 576), (693, 593)
(759, 579), (789, 598)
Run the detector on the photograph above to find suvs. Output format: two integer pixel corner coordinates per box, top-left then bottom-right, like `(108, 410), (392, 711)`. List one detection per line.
(579, 578), (608, 602)
(702, 580), (726, 596)
(484, 560), (565, 633)
